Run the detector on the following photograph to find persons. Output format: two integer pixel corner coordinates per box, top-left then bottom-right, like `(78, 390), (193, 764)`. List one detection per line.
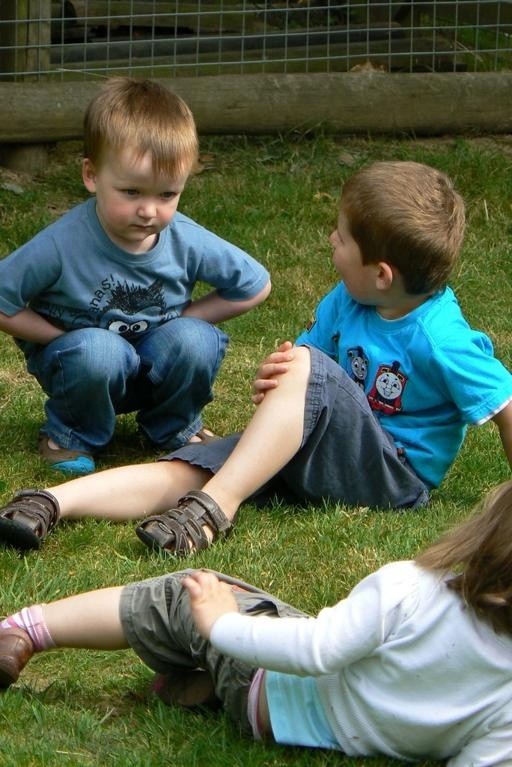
(0, 158), (512, 559)
(0, 476), (512, 767)
(0, 73), (273, 476)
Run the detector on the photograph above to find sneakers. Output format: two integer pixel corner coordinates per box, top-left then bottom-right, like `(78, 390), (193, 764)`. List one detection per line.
(157, 669), (214, 708)
(185, 429), (219, 446)
(0, 625), (35, 689)
(40, 436), (96, 473)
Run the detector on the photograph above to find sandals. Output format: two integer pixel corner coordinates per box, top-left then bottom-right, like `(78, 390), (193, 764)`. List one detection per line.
(136, 490), (234, 557)
(0, 487), (60, 550)
